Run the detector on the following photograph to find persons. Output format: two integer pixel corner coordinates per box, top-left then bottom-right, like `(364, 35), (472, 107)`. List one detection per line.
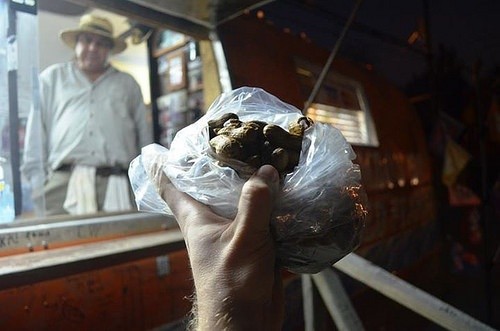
(148, 162), (303, 331)
(21, 20), (153, 219)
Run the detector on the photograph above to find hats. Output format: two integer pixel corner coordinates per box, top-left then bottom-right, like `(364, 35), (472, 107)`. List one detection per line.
(59, 15), (127, 55)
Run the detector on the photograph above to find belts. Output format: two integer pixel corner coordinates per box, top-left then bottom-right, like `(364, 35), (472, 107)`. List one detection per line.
(58, 163), (127, 176)
